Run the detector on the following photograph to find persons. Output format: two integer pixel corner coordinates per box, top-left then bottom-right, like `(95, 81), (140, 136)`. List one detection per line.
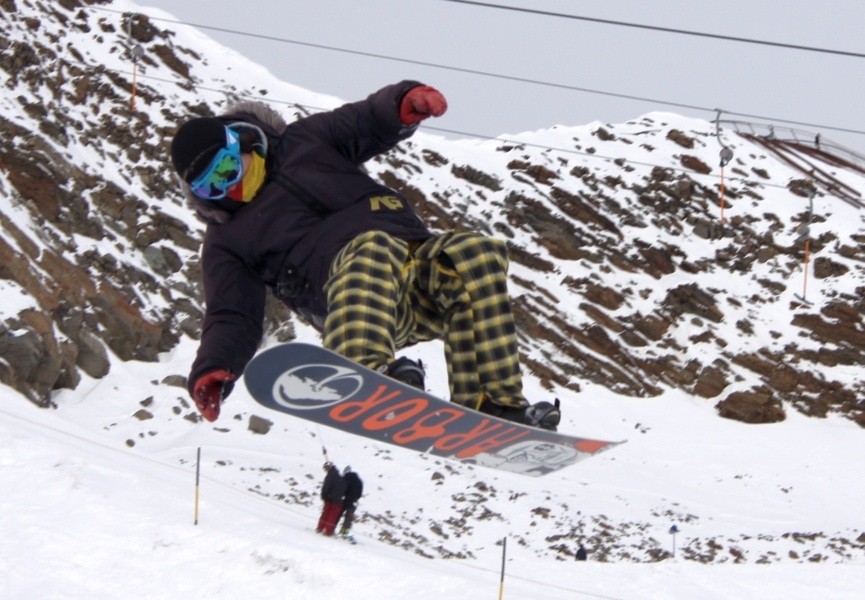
(171, 80), (560, 430)
(332, 465), (365, 539)
(317, 461), (347, 535)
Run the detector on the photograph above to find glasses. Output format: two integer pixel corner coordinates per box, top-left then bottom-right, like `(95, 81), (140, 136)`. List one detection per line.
(190, 149), (242, 200)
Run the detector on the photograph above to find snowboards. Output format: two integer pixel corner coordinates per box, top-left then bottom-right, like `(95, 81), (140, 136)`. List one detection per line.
(244, 342), (628, 477)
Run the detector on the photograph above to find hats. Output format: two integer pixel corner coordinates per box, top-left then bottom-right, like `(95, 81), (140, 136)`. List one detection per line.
(345, 466), (351, 473)
(324, 462), (333, 470)
(171, 117), (252, 212)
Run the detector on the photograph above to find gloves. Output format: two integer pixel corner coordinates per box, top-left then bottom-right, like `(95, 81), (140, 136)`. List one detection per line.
(192, 368), (235, 422)
(400, 85), (448, 126)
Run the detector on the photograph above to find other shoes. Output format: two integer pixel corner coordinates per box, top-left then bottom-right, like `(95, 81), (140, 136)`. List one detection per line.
(478, 399), (559, 430)
(388, 358), (425, 392)
